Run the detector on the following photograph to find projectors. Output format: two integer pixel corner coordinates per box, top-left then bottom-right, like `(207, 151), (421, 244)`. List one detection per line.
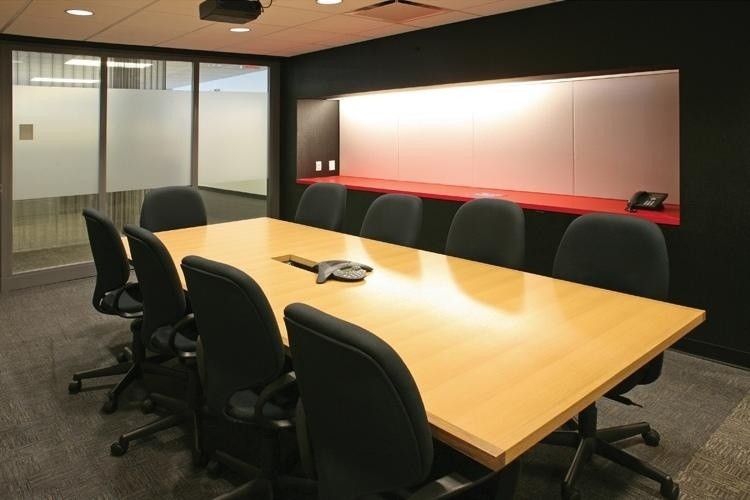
(199, 0), (261, 24)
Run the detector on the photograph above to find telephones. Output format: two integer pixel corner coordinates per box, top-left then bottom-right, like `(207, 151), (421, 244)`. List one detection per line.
(315, 260), (374, 284)
(627, 191), (668, 209)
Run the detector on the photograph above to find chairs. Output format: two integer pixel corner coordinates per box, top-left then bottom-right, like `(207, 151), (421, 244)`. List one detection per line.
(525, 211), (683, 499)
(359, 191), (426, 247)
(275, 291), (520, 500)
(177, 252), (307, 500)
(112, 223), (212, 473)
(70, 206), (174, 416)
(444, 198), (529, 272)
(138, 187), (209, 236)
(295, 181), (350, 231)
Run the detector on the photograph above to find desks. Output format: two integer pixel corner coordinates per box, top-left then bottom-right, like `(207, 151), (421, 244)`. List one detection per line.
(119, 212), (708, 473)
